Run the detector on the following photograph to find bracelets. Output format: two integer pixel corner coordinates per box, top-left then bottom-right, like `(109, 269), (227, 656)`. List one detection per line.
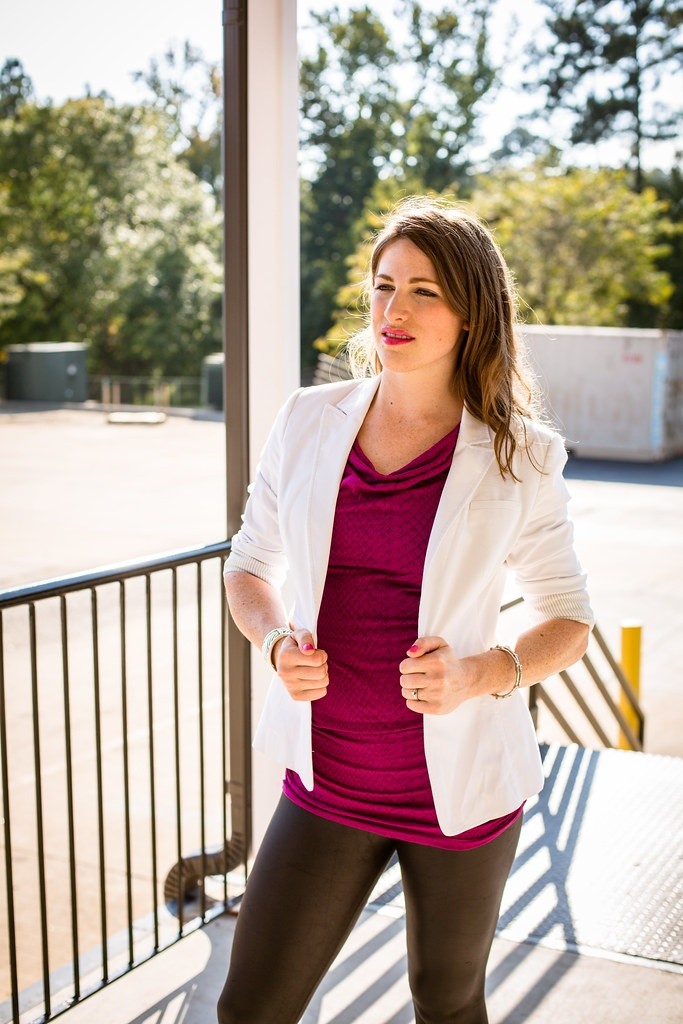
(262, 627), (294, 672)
(488, 645), (522, 699)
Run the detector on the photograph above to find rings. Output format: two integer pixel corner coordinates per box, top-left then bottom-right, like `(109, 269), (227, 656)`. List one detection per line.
(413, 688), (419, 701)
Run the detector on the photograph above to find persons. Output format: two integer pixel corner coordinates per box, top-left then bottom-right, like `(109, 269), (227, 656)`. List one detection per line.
(217, 194), (596, 1023)
